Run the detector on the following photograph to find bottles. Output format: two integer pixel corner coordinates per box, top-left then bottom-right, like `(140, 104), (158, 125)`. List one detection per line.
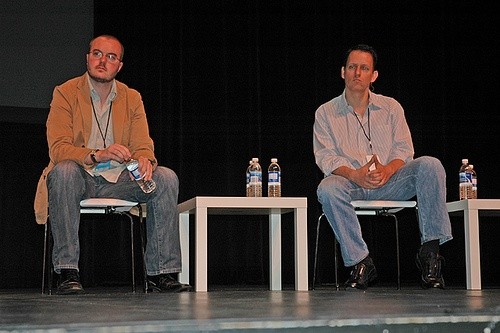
(459, 159), (472, 200)
(246, 160), (253, 196)
(249, 158), (262, 197)
(268, 158), (281, 197)
(469, 165), (477, 199)
(125, 158), (156, 193)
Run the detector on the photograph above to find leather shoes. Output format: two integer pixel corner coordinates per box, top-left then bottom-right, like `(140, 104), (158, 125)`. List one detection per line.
(144, 273), (192, 294)
(56, 270), (83, 295)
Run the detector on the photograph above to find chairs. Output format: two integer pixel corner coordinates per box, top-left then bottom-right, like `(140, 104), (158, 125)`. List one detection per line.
(41, 198), (153, 295)
(311, 175), (445, 291)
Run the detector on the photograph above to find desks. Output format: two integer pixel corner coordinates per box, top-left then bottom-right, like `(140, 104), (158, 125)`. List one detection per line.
(178, 196), (308, 292)
(447, 199), (500, 291)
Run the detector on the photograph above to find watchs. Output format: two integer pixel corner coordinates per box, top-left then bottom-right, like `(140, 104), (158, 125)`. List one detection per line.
(91, 149), (100, 165)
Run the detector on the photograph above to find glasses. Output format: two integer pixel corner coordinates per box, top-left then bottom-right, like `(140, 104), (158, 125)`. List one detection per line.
(88, 50), (122, 63)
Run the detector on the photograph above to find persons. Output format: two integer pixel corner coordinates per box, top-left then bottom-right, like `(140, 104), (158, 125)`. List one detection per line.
(34, 34), (193, 294)
(313, 45), (454, 292)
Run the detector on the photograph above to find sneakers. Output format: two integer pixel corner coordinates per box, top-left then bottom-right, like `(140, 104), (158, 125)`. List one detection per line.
(415, 245), (445, 291)
(344, 256), (378, 291)
